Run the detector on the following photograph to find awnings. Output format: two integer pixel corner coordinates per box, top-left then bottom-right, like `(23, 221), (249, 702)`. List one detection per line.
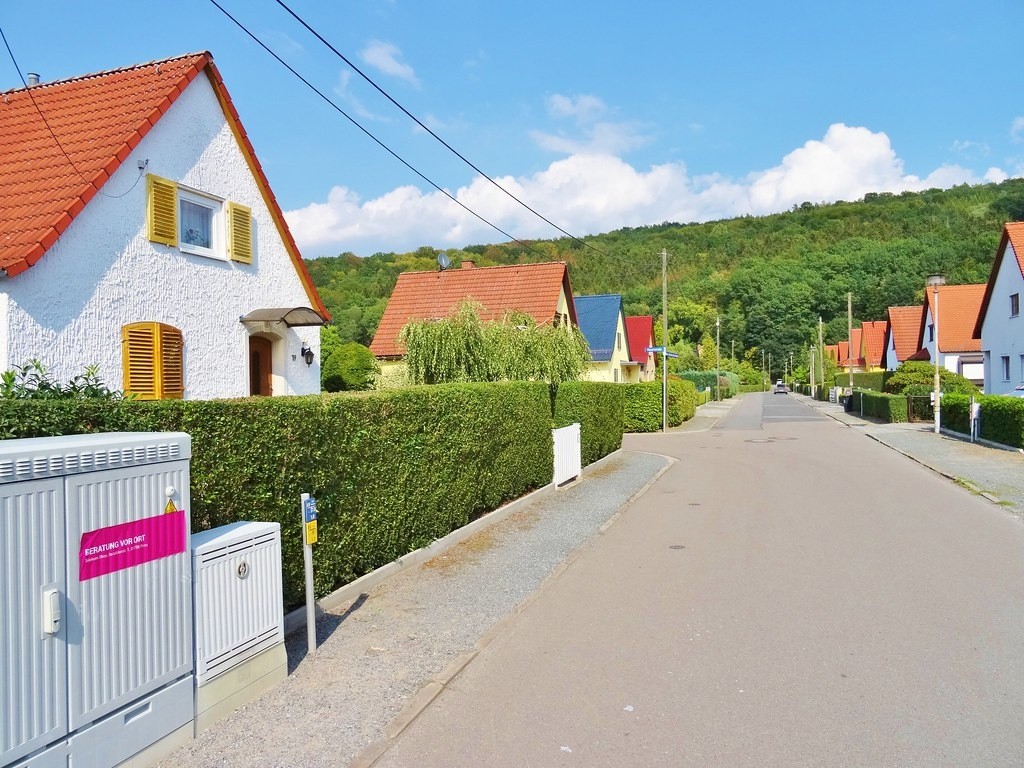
(241, 307), (325, 328)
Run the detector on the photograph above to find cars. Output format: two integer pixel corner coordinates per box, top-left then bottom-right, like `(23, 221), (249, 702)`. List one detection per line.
(774, 383), (788, 394)
(776, 379), (782, 384)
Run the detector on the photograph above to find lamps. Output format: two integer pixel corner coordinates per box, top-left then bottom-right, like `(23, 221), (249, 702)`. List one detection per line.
(301, 340), (314, 367)
